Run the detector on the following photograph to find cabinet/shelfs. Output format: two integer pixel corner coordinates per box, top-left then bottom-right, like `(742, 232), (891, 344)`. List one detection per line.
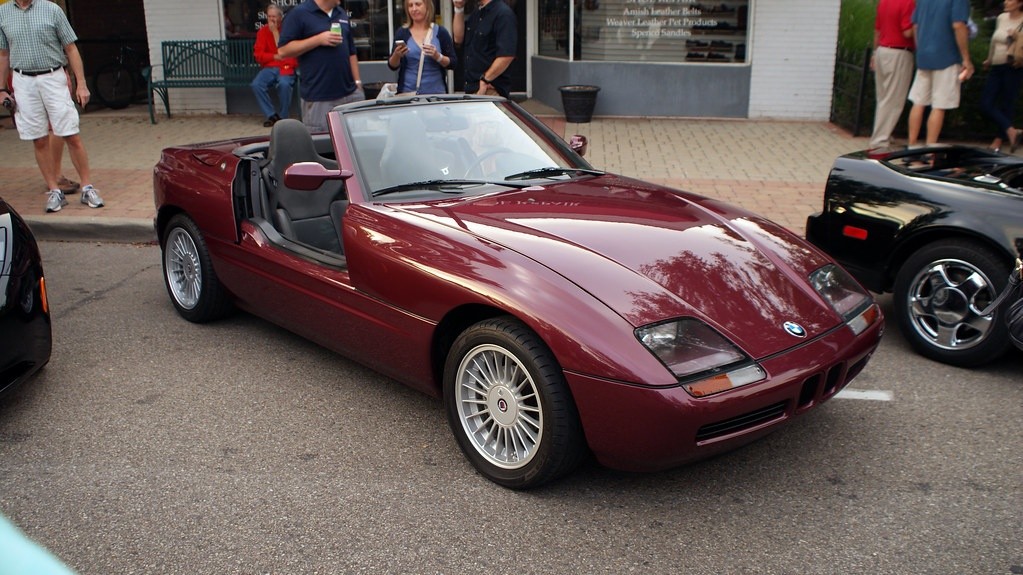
(685, 10), (736, 62)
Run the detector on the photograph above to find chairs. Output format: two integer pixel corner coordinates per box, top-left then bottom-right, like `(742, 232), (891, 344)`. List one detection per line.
(260, 117), (352, 254)
(380, 109), (446, 190)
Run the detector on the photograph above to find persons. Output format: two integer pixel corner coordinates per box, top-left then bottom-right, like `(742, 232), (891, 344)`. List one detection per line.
(8, 69), (80, 195)
(278, 0), (365, 131)
(387, 0), (457, 95)
(453, 0), (517, 98)
(983, 0), (1023, 154)
(0, 0), (104, 212)
(224, 0), (265, 39)
(907, 0), (974, 143)
(870, 0), (915, 147)
(251, 5), (298, 127)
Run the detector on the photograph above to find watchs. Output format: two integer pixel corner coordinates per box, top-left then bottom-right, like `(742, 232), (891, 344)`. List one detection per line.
(480, 75), (489, 84)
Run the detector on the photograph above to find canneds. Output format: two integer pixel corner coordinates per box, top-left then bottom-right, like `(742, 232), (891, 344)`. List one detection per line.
(330, 23), (342, 36)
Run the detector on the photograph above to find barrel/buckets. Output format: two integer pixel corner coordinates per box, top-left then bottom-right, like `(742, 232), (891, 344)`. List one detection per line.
(559, 85), (601, 122)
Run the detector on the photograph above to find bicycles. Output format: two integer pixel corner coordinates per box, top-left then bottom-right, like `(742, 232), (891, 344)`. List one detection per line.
(91, 33), (153, 111)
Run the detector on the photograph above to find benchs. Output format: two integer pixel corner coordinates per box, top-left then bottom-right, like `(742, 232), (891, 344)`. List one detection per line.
(146, 40), (303, 124)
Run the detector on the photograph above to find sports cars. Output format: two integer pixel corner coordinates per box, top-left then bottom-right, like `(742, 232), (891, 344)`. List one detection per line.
(804, 141), (1021, 365)
(149, 93), (886, 492)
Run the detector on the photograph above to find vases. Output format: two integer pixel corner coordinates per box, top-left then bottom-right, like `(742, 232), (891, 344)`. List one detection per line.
(558, 84), (600, 122)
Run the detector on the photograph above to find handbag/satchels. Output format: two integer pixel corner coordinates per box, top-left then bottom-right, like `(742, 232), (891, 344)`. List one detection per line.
(377, 82), (398, 98)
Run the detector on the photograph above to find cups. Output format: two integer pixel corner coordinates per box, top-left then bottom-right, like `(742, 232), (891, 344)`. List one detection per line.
(331, 23), (342, 35)
(958, 68), (968, 83)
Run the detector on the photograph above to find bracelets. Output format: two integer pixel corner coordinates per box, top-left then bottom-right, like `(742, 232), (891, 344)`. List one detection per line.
(0, 89), (6, 92)
(436, 53), (443, 62)
(355, 80), (362, 83)
(454, 7), (464, 13)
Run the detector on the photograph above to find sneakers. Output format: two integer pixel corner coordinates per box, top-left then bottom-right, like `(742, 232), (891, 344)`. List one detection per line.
(81, 185), (104, 207)
(45, 190), (67, 212)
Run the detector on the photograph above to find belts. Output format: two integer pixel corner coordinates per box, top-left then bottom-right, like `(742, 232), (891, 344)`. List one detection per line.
(891, 47), (912, 51)
(14, 65), (61, 76)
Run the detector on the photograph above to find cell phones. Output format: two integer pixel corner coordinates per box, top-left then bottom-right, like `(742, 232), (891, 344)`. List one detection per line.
(396, 40), (407, 48)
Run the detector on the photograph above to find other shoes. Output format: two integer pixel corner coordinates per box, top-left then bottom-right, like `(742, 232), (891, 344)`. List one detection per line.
(58, 182), (76, 194)
(58, 176), (80, 189)
(686, 4), (735, 60)
(1010, 129), (1023, 153)
(264, 114), (279, 126)
